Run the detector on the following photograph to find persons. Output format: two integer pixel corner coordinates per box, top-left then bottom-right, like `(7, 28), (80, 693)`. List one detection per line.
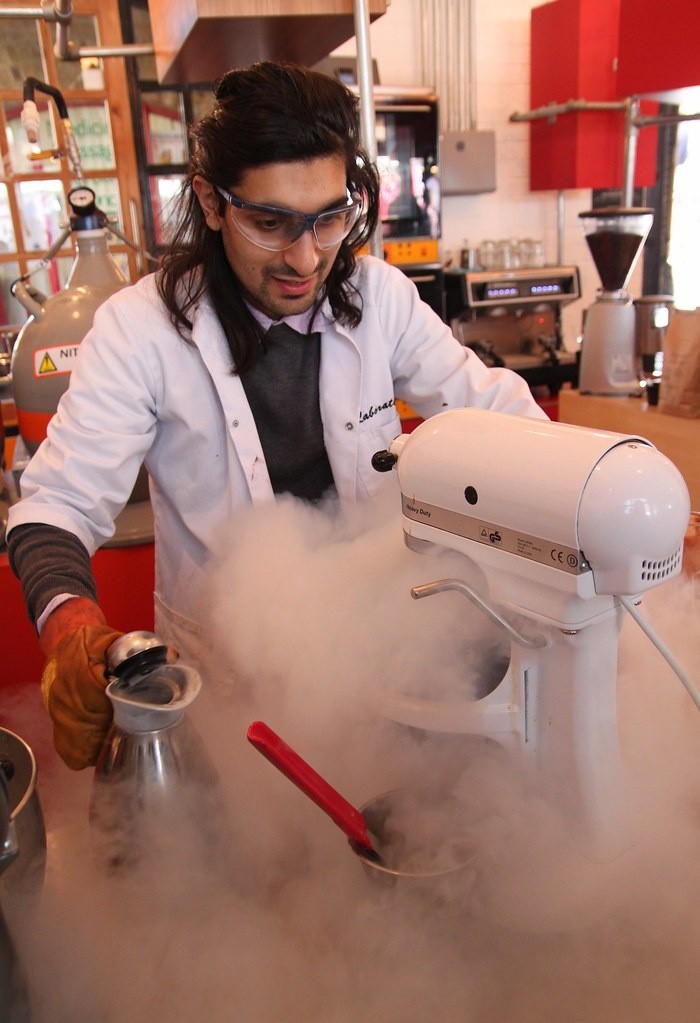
(3, 61), (555, 773)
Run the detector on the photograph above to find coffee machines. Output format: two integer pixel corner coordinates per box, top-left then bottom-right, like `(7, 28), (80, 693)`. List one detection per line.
(578, 208), (658, 398)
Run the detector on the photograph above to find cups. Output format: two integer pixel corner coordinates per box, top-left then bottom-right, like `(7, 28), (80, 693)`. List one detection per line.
(341, 785), (487, 919)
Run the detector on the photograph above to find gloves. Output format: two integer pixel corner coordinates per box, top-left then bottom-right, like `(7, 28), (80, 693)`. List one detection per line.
(39, 597), (181, 771)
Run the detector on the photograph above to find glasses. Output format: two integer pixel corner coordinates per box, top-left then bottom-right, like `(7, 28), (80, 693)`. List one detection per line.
(205, 174), (365, 254)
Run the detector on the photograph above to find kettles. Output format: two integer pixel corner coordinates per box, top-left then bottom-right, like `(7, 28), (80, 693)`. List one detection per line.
(89, 632), (229, 936)
